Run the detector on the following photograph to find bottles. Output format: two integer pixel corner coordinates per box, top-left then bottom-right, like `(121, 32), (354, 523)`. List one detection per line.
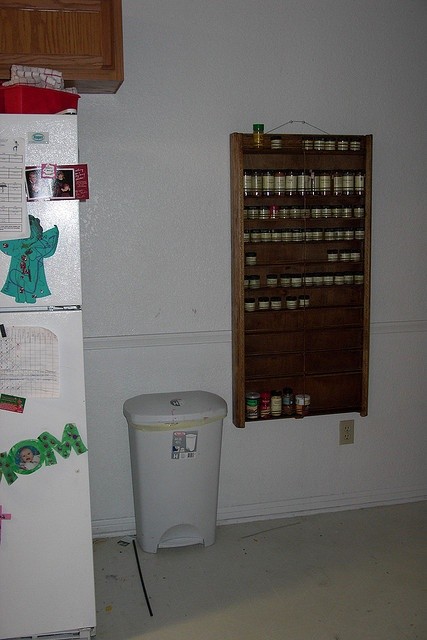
(260, 393), (271, 419)
(253, 123), (264, 150)
(319, 169), (332, 196)
(262, 168), (275, 196)
(242, 169), (252, 197)
(309, 169), (320, 196)
(355, 169), (366, 196)
(283, 388), (295, 415)
(251, 168), (263, 196)
(274, 168), (286, 196)
(270, 389), (283, 417)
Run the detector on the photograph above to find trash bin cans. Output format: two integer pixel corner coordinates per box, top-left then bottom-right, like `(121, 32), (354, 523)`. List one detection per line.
(122, 390), (227, 553)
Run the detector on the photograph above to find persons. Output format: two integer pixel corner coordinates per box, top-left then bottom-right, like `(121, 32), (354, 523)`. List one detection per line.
(53, 171), (71, 197)
(18, 445), (42, 470)
(28, 171), (46, 197)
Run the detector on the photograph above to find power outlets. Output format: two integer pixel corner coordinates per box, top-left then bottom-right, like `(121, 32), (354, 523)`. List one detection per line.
(339, 420), (354, 445)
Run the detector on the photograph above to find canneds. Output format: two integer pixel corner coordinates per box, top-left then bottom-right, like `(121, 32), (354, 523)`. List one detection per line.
(242, 171), (251, 197)
(307, 168), (320, 195)
(354, 168), (365, 196)
(261, 171), (273, 197)
(273, 170), (285, 197)
(319, 168), (331, 196)
(250, 171), (261, 197)
(259, 394), (271, 419)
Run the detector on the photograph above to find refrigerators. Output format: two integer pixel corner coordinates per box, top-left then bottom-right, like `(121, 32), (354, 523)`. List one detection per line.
(0, 109), (97, 640)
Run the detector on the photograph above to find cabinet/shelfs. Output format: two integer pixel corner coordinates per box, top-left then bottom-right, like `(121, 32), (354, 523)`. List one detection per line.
(231, 132), (372, 427)
(0, 1), (124, 94)
